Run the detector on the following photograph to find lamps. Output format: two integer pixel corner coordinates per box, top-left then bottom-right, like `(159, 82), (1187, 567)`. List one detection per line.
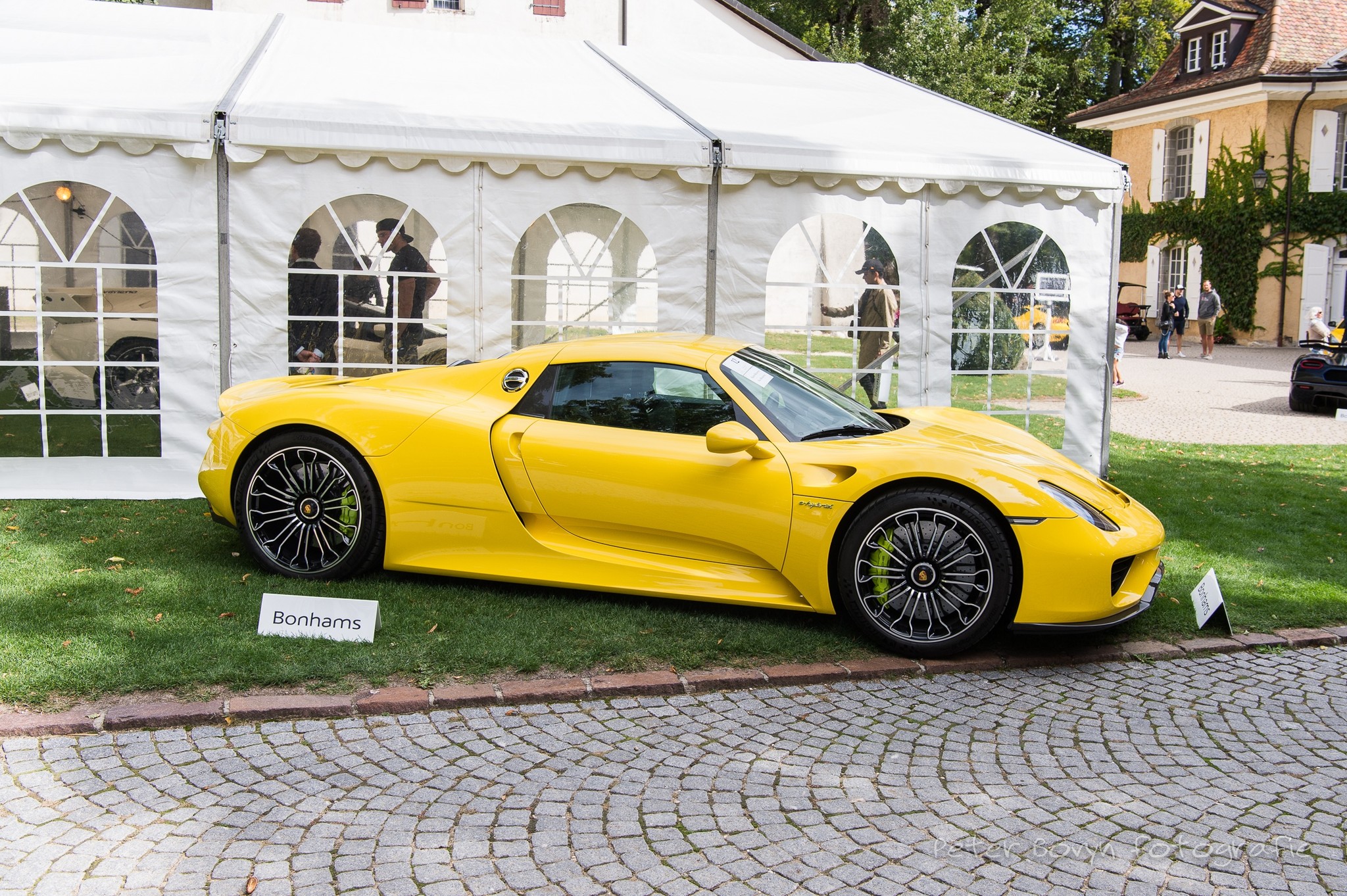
(1251, 169), (1270, 189)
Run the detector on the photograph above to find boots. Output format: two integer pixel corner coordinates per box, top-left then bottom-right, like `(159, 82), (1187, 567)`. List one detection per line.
(1158, 352), (1171, 359)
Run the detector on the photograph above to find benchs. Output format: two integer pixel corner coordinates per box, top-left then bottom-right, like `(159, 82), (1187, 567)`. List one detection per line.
(1117, 302), (1145, 325)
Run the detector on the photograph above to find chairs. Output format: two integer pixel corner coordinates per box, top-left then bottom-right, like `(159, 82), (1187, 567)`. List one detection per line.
(554, 364), (676, 433)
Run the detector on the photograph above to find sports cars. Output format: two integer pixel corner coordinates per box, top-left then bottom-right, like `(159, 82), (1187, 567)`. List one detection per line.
(33, 281), (454, 420)
(198, 328), (1170, 662)
(1011, 303), (1072, 349)
(1288, 339), (1347, 413)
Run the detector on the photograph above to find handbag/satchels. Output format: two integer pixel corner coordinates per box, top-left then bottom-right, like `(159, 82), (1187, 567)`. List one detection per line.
(847, 318), (862, 340)
(1159, 319), (1174, 336)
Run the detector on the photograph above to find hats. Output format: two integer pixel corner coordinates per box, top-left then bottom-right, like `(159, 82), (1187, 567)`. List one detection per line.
(855, 258), (884, 275)
(1174, 284), (1186, 290)
(375, 218), (414, 244)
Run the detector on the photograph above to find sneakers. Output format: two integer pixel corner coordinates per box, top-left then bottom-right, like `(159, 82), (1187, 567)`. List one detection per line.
(1195, 353), (1213, 360)
(1166, 351), (1186, 358)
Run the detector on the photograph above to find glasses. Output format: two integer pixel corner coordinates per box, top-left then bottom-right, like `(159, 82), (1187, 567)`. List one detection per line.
(1318, 311), (1323, 314)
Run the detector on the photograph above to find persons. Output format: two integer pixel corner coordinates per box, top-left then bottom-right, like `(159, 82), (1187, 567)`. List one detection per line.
(1166, 285), (1190, 358)
(373, 218), (440, 373)
(1113, 318), (1129, 387)
(340, 254), (384, 341)
(283, 227), (343, 378)
(1156, 289), (1177, 359)
(1308, 306), (1331, 354)
(819, 256), (899, 408)
(1197, 279), (1221, 359)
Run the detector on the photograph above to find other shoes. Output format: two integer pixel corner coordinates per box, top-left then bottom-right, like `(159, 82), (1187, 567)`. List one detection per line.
(1112, 379), (1124, 386)
